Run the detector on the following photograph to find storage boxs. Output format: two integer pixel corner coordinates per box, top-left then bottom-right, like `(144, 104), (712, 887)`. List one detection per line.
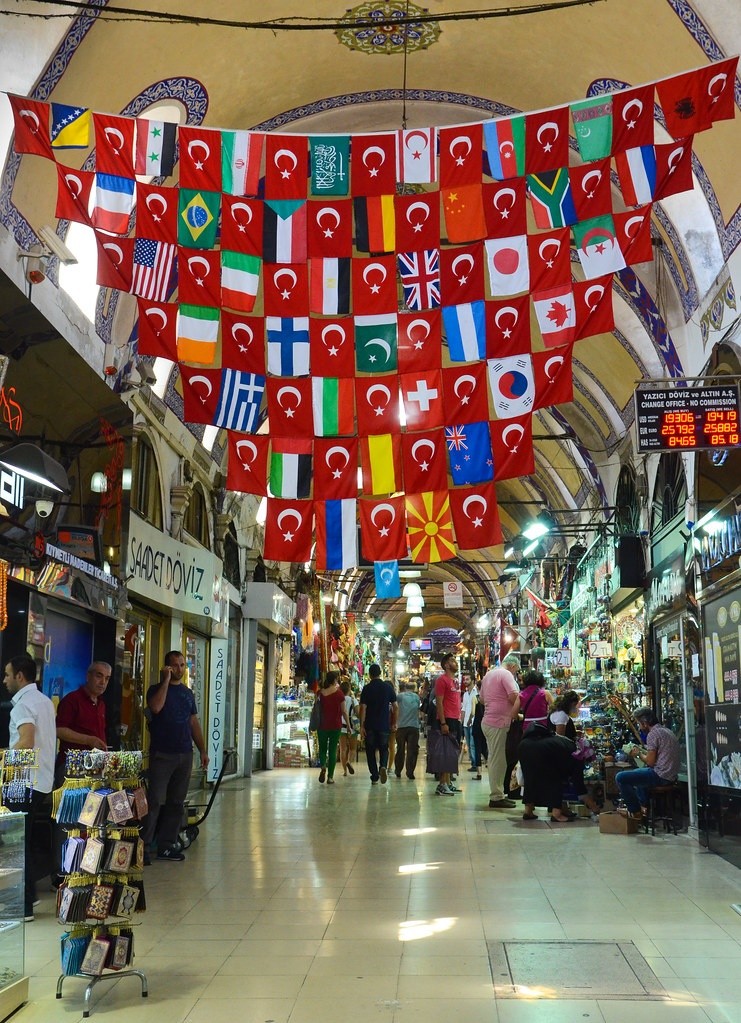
(604, 761), (633, 794)
(599, 811), (639, 835)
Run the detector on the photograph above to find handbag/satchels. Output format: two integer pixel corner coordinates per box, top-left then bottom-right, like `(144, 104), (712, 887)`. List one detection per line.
(426, 730), (462, 777)
(309, 688), (321, 731)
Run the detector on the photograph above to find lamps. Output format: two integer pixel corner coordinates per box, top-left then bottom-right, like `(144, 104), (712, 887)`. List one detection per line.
(402, 578), (425, 629)
(25, 244), (46, 285)
(0, 442), (72, 498)
(497, 506), (616, 586)
(334, 585), (349, 596)
(333, 609), (393, 644)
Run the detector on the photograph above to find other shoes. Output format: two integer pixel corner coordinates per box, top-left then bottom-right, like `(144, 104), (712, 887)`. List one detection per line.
(379, 767), (387, 784)
(319, 768), (326, 783)
(346, 761), (354, 774)
(523, 813), (538, 820)
(505, 794), (523, 800)
(327, 780), (334, 784)
(489, 799), (516, 808)
(0, 906), (34, 921)
(472, 774), (482, 780)
(49, 876), (64, 893)
(435, 781), (462, 796)
(550, 815), (573, 822)
(467, 767), (476, 772)
(387, 768), (394, 775)
(33, 895), (42, 907)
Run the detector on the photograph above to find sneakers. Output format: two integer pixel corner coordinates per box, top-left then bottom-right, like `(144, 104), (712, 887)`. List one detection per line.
(156, 846), (185, 861)
(640, 806), (648, 817)
(617, 808), (643, 821)
(143, 851), (152, 866)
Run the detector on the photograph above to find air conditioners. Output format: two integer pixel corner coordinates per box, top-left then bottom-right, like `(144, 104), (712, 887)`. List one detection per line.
(103, 343), (119, 375)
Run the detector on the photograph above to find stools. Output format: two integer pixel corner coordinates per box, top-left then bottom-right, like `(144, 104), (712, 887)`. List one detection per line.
(645, 783), (677, 837)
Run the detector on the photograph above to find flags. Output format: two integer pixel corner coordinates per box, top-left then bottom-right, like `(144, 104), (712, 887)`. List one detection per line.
(5, 54), (739, 601)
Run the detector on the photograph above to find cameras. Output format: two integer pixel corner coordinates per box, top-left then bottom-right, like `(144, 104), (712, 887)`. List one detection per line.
(137, 364), (157, 386)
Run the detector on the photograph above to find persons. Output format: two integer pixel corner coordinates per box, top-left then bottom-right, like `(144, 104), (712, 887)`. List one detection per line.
(52, 661), (111, 894)
(2, 655), (56, 923)
(615, 707), (680, 820)
(314, 652), (600, 823)
(143, 649), (209, 868)
